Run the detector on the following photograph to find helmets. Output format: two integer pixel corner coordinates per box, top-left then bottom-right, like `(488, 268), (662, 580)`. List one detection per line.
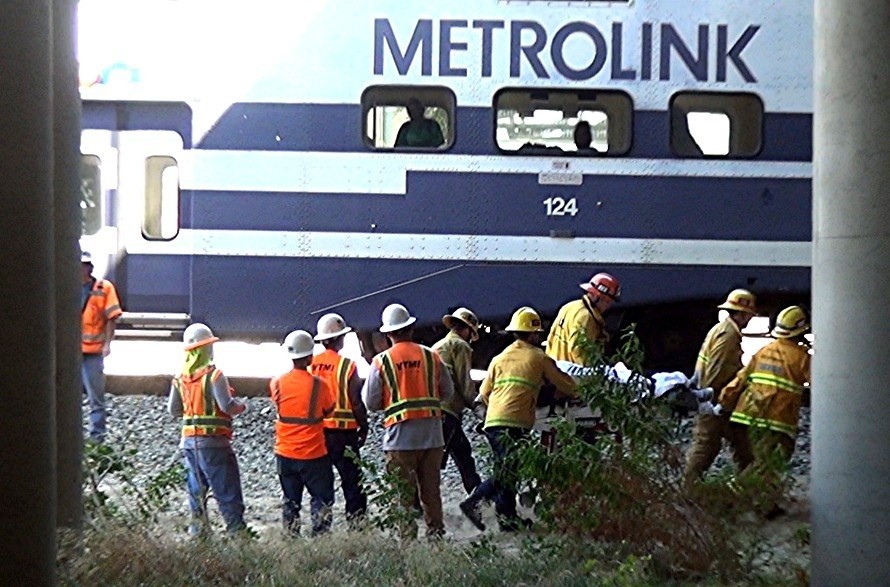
(184, 324), (219, 351)
(285, 329), (315, 359)
(314, 313), (351, 341)
(772, 306), (809, 339)
(442, 308), (479, 341)
(717, 289), (758, 315)
(82, 250), (92, 263)
(380, 303), (416, 332)
(580, 273), (621, 303)
(504, 307), (544, 332)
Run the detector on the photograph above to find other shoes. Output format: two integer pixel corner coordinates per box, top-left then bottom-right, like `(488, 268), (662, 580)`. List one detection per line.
(500, 518), (534, 532)
(458, 502), (486, 531)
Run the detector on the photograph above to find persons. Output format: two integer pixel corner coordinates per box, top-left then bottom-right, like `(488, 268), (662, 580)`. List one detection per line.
(270, 329), (338, 540)
(556, 360), (713, 403)
(362, 302), (455, 543)
(81, 251), (123, 448)
(459, 307), (578, 530)
(680, 289), (758, 489)
(310, 313), (370, 532)
(394, 98), (445, 148)
(574, 121), (598, 152)
(544, 272), (623, 445)
(714, 304), (810, 520)
(430, 307), (494, 509)
(168, 323), (261, 540)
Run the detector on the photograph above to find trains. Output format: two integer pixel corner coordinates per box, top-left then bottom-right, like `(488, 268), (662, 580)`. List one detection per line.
(73, 1), (814, 373)
